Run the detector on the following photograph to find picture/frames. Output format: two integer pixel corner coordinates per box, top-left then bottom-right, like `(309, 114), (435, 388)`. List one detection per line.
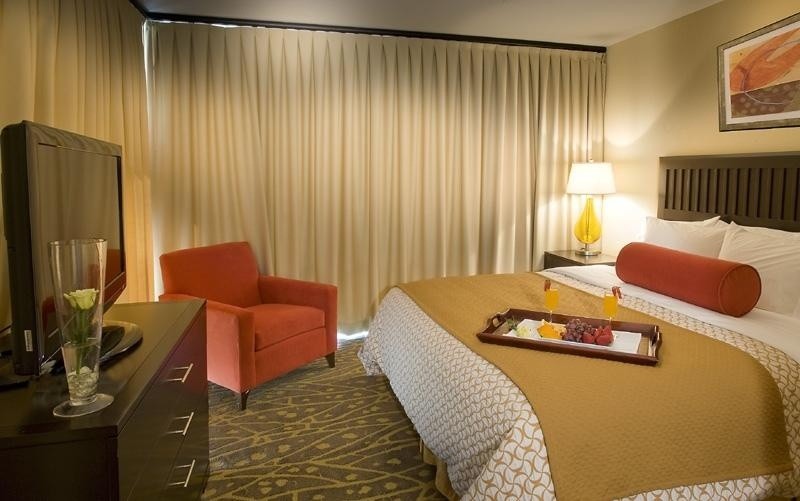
(716, 13), (800, 132)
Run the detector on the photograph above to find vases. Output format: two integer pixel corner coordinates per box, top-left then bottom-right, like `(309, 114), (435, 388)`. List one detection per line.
(48, 237), (115, 417)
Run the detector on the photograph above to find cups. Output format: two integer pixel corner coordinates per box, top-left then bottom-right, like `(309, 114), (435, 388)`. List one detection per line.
(604, 291), (618, 331)
(544, 283), (559, 329)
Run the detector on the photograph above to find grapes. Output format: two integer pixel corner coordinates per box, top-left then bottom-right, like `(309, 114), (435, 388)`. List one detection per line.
(561, 318), (596, 343)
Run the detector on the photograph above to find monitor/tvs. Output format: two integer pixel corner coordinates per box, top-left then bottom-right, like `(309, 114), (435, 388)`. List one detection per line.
(0, 119), (144, 391)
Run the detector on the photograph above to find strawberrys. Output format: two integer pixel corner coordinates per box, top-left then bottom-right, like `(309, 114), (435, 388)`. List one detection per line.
(594, 326), (602, 333)
(597, 335), (609, 346)
(604, 325), (613, 342)
(594, 331), (601, 342)
(582, 332), (594, 344)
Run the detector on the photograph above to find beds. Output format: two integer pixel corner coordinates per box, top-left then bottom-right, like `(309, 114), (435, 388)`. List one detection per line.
(355, 151), (800, 501)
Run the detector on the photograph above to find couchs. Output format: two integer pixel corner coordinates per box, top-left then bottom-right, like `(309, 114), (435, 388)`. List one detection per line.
(159, 240), (338, 412)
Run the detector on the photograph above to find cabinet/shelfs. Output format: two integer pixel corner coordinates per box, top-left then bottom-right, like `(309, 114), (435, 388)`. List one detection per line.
(0, 298), (210, 501)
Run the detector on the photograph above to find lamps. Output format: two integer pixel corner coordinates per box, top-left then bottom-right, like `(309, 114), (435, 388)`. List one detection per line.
(566, 161), (616, 256)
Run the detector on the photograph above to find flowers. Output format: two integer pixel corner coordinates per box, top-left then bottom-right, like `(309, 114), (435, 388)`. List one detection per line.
(62, 288), (99, 374)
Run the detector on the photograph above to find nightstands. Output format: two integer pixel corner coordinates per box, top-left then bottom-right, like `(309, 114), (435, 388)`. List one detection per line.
(543, 249), (617, 271)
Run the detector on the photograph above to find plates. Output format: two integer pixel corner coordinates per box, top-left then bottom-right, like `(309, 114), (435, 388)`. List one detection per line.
(476, 309), (663, 366)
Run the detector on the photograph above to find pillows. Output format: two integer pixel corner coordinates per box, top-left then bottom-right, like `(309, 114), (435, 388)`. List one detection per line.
(643, 215), (722, 258)
(717, 221), (800, 318)
(615, 241), (762, 318)
